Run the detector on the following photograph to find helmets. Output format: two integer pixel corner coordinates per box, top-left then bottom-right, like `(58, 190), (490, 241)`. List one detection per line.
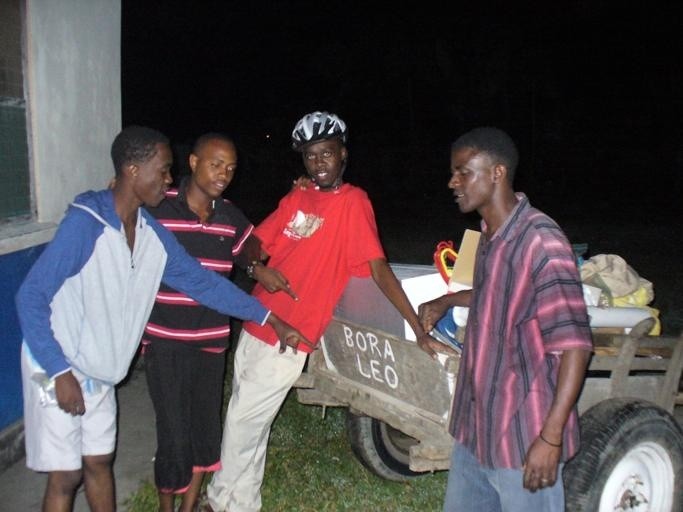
(291, 112), (346, 151)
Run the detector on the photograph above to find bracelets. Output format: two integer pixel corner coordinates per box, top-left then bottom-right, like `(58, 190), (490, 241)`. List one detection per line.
(541, 431), (563, 447)
(247, 260), (263, 280)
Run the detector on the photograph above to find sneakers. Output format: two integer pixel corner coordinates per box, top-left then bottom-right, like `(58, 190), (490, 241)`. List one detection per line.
(191, 491), (213, 512)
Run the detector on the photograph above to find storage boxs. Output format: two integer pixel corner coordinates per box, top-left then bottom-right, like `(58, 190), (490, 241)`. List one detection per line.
(432, 228), (482, 355)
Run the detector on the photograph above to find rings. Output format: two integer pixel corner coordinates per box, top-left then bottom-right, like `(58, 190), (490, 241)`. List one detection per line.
(541, 478), (548, 483)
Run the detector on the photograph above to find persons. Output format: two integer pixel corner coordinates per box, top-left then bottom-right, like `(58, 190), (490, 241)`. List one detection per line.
(188, 110), (463, 512)
(14, 127), (320, 512)
(417, 127), (595, 512)
(108, 132), (298, 512)
